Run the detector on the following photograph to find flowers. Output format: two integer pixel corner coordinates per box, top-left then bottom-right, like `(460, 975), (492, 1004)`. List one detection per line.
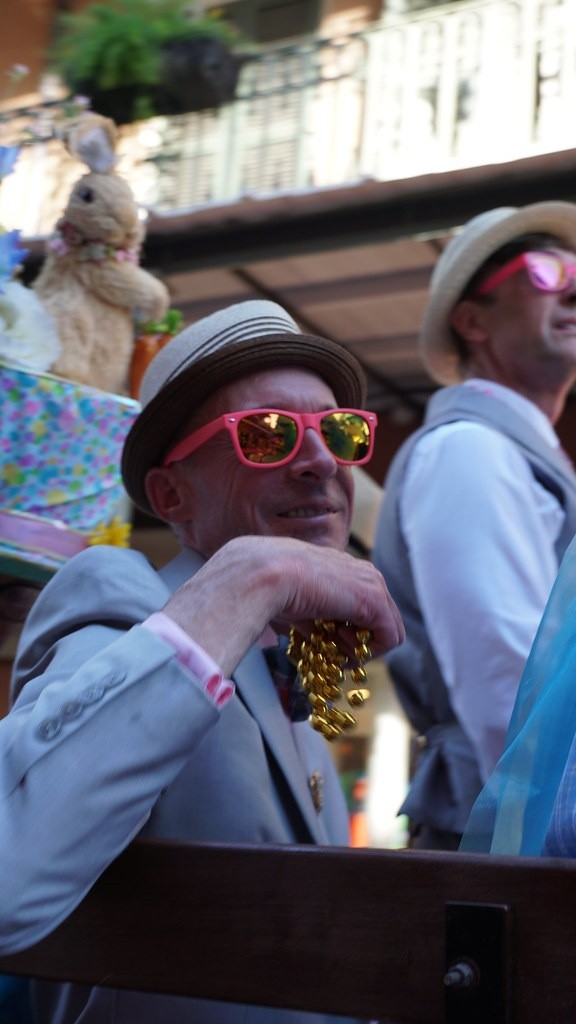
(88, 512), (133, 548)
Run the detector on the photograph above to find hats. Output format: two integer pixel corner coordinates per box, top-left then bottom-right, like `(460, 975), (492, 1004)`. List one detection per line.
(120, 298), (366, 516)
(418, 201), (576, 387)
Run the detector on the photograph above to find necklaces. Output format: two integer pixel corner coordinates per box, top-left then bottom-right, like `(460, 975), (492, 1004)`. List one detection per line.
(286, 616), (370, 739)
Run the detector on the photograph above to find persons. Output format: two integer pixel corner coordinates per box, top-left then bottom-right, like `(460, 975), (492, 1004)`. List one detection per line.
(0, 300), (407, 958)
(369, 199), (576, 861)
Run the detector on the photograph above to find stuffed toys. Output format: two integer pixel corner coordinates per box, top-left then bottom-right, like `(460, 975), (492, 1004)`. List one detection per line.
(25, 111), (167, 398)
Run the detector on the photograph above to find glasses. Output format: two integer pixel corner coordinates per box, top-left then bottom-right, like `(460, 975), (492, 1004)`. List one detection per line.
(480, 251), (575, 295)
(159, 409), (377, 469)
